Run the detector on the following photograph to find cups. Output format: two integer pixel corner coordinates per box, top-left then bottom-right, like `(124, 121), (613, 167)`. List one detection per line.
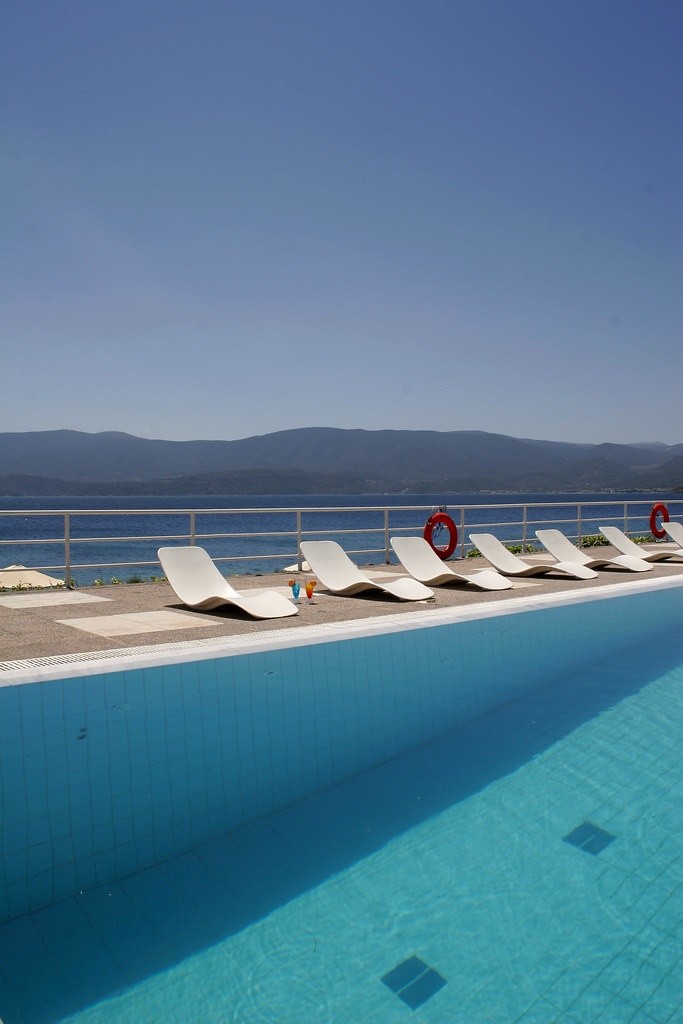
(291, 580), (302, 604)
(304, 579), (314, 604)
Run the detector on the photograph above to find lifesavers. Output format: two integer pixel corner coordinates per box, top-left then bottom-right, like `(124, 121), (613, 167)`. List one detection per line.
(423, 512), (459, 560)
(649, 503), (670, 538)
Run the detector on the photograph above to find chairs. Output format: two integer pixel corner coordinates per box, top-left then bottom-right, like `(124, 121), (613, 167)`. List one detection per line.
(599, 525), (683, 564)
(298, 541), (437, 602)
(469, 530), (602, 581)
(157, 542), (301, 622)
(661, 522), (683, 549)
(534, 528), (653, 573)
(389, 535), (515, 593)
